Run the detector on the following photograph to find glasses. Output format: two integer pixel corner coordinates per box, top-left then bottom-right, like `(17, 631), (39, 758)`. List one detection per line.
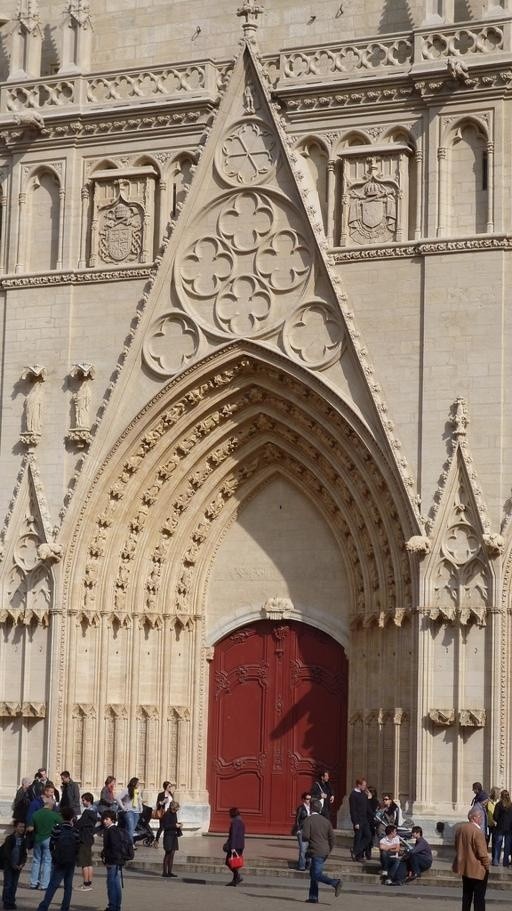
(305, 798), (311, 800)
(382, 798), (390, 800)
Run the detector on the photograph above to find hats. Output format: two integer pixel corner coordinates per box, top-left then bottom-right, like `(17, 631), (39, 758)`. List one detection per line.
(477, 791), (489, 801)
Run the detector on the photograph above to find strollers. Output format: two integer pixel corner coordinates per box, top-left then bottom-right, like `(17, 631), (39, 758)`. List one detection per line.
(373, 811), (415, 853)
(133, 802), (159, 847)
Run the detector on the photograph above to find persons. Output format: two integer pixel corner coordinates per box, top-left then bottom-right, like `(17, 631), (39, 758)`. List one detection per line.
(292, 770), (512, 911)
(223, 807), (246, 886)
(0, 767), (183, 911)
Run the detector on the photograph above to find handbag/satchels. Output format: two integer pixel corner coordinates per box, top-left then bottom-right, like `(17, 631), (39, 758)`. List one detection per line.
(226, 848), (244, 871)
(175, 822), (183, 837)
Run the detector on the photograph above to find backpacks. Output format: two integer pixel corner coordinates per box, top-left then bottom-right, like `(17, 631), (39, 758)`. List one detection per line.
(109, 826), (135, 862)
(53, 823), (78, 867)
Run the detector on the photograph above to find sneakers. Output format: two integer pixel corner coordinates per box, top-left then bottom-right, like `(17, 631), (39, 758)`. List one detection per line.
(356, 855), (366, 863)
(303, 898), (319, 904)
(161, 872), (178, 877)
(408, 871), (420, 880)
(334, 878), (343, 897)
(224, 878), (244, 887)
(382, 869), (394, 885)
(297, 864), (306, 871)
(72, 881), (93, 892)
